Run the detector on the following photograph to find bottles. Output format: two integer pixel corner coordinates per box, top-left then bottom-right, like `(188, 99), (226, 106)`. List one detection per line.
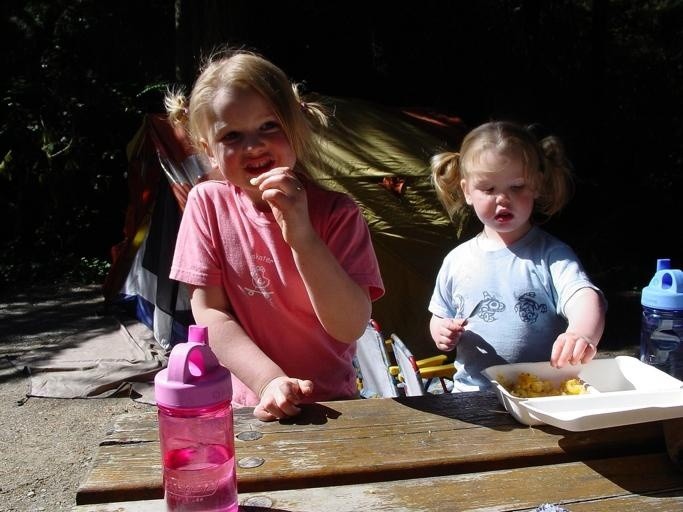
(151, 325), (238, 511)
(641, 258), (683, 382)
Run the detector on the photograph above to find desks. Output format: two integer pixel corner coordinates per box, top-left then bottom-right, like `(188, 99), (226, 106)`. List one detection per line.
(72, 390), (683, 511)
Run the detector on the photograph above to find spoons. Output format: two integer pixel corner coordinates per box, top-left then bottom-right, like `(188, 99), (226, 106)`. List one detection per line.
(460, 300), (484, 328)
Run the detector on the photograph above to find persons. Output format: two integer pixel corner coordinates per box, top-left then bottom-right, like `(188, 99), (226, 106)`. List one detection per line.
(168, 53), (386, 423)
(425, 121), (606, 394)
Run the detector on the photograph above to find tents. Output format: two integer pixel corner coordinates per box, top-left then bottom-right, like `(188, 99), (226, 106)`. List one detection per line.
(95, 93), (477, 366)
